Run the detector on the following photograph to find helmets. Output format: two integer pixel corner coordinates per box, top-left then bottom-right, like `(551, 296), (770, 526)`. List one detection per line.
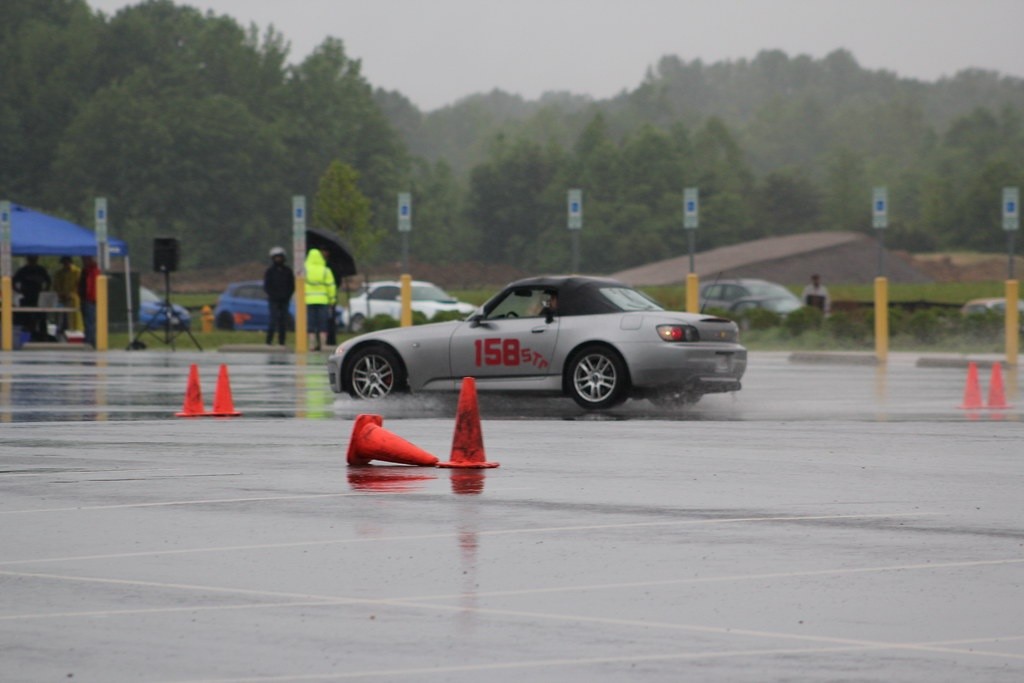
(270, 246), (286, 256)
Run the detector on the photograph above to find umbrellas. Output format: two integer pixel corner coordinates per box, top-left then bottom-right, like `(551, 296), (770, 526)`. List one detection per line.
(305, 228), (357, 277)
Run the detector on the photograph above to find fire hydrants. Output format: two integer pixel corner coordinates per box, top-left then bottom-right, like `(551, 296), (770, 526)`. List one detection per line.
(200, 305), (215, 333)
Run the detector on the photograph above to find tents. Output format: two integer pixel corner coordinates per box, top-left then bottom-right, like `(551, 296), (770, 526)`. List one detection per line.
(0, 199), (146, 351)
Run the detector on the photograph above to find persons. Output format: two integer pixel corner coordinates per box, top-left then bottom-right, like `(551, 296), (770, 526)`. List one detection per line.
(538, 294), (556, 315)
(802, 273), (831, 314)
(304, 248), (337, 350)
(12, 255), (51, 306)
(77, 255), (100, 344)
(320, 245), (342, 345)
(263, 246), (295, 347)
(52, 256), (84, 331)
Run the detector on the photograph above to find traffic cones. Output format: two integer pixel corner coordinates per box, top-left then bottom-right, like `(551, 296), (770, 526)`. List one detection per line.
(204, 363), (242, 417)
(982, 362), (1015, 410)
(344, 412), (440, 467)
(955, 362), (988, 410)
(175, 363), (210, 417)
(433, 375), (501, 470)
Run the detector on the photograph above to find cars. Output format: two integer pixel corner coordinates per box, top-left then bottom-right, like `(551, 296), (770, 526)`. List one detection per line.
(211, 278), (347, 336)
(698, 278), (806, 322)
(958, 296), (1024, 319)
(339, 278), (480, 336)
(137, 284), (193, 330)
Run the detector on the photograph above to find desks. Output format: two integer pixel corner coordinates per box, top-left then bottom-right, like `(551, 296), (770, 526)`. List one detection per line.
(11, 306), (75, 343)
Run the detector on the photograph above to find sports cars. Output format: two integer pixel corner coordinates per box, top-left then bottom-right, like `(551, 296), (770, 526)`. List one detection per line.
(325, 273), (748, 411)
